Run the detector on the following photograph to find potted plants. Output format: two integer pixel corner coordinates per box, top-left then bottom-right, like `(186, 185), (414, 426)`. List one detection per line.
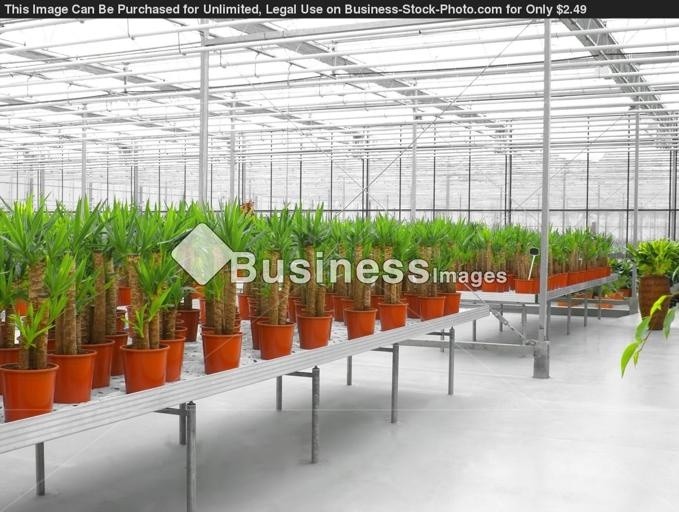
(625, 236), (679, 330)
(0, 191), (631, 425)
